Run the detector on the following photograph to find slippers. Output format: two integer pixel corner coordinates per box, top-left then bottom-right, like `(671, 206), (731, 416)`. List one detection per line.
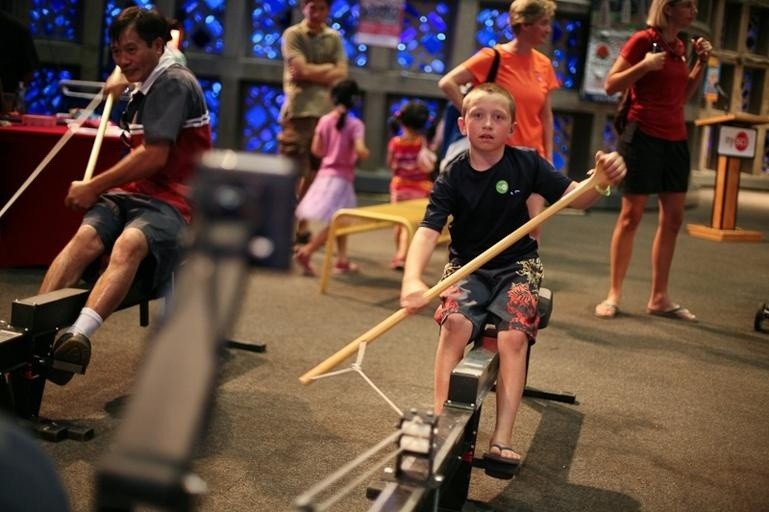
(646, 304), (699, 323)
(482, 444), (519, 479)
(594, 301), (619, 319)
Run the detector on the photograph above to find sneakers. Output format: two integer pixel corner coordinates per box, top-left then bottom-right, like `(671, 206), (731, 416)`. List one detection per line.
(334, 260), (358, 274)
(294, 249), (315, 275)
(47, 332), (91, 385)
(390, 255), (407, 269)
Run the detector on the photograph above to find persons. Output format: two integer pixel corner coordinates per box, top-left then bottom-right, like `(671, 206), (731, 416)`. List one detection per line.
(388, 102), (438, 270)
(593, 0), (713, 323)
(293, 78), (369, 277)
(437, 0), (563, 252)
(35, 6), (213, 386)
(431, 82), (469, 175)
(399, 83), (628, 467)
(278, 1), (348, 248)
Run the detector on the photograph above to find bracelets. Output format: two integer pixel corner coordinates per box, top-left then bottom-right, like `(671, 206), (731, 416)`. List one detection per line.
(698, 58), (707, 64)
(587, 167), (611, 197)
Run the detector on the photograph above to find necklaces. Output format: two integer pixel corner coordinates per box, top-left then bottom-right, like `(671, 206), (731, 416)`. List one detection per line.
(657, 31), (680, 52)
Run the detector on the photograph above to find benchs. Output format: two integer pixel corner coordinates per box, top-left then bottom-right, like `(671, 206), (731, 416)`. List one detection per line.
(320, 197), (454, 294)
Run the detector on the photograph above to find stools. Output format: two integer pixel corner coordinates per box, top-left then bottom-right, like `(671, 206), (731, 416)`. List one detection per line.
(478, 287), (553, 337)
(82, 248), (190, 327)
